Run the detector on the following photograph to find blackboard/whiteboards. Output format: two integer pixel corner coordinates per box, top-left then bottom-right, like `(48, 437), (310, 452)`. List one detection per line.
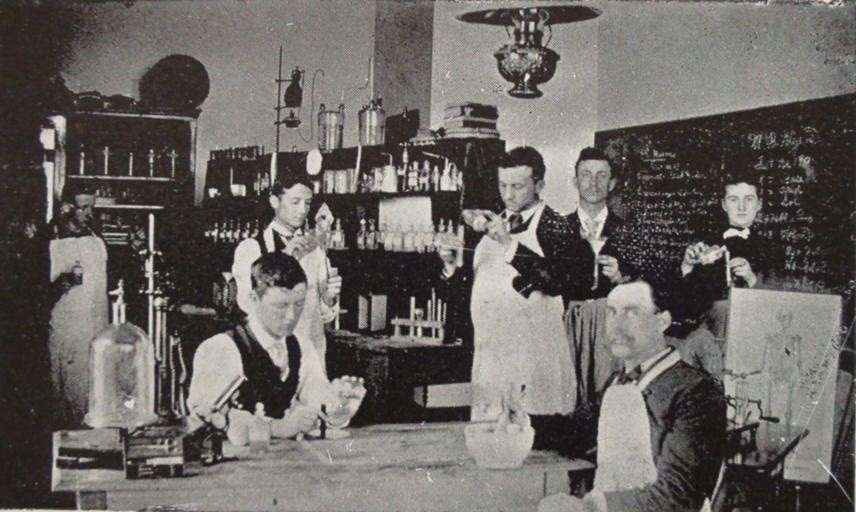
(594, 93), (856, 353)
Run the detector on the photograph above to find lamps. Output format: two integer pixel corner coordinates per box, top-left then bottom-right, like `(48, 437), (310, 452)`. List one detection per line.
(457, 1), (601, 99)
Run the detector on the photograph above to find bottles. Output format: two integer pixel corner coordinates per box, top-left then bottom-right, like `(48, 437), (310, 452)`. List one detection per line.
(247, 401), (274, 454)
(355, 219), (456, 254)
(698, 243), (727, 266)
(359, 160), (463, 193)
(253, 172), (271, 203)
(202, 218), (262, 245)
(304, 216), (346, 251)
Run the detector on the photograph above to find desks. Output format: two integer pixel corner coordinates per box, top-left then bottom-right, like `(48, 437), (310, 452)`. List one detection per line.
(77, 424), (596, 512)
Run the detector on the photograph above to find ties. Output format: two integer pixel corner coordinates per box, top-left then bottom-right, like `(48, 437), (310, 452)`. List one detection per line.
(507, 212), (523, 233)
(586, 218), (600, 242)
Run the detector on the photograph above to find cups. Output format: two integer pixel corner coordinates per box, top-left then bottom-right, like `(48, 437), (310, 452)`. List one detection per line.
(586, 233), (609, 256)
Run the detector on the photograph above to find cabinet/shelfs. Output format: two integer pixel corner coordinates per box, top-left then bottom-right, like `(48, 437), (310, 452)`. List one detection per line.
(65, 109), (196, 245)
(185, 142), (505, 422)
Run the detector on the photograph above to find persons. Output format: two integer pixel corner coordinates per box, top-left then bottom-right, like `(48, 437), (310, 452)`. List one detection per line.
(668, 168), (784, 384)
(433, 141), (598, 457)
(183, 250), (367, 444)
(22, 179), (115, 435)
(232, 171), (345, 380)
(557, 145), (643, 421)
(489, 273), (729, 511)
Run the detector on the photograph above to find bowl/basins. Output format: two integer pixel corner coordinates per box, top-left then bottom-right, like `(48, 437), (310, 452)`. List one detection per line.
(464, 422), (536, 469)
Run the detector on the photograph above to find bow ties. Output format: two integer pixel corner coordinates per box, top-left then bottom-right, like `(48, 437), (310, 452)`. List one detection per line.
(616, 367), (641, 387)
(722, 227), (751, 242)
